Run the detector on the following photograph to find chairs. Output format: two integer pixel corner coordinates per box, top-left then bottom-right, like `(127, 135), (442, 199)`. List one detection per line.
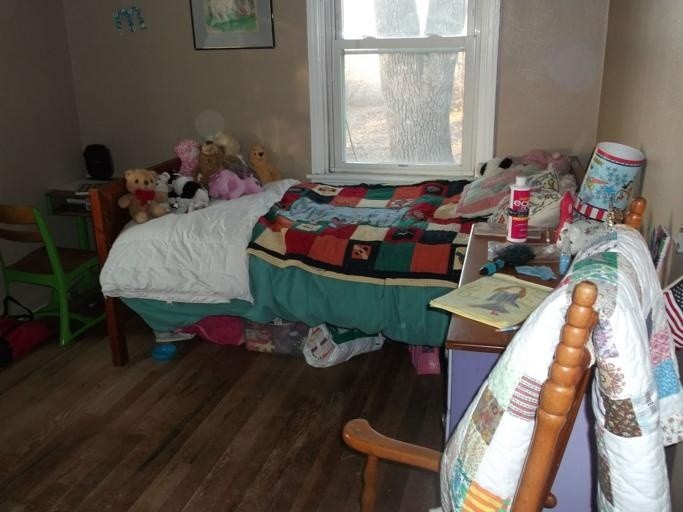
(0, 204), (108, 350)
(88, 157), (583, 369)
(340, 194), (648, 508)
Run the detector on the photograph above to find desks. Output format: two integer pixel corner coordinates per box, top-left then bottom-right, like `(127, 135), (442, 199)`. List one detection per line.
(46, 178), (123, 311)
(439, 221), (600, 510)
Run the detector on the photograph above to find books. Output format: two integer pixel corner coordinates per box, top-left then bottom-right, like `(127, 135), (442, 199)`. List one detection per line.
(474, 222), (541, 240)
(429, 276), (555, 329)
(651, 224), (671, 270)
(47, 179), (111, 211)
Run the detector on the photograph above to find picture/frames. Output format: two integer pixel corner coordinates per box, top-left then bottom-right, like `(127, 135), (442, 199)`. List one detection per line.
(188, 1), (275, 52)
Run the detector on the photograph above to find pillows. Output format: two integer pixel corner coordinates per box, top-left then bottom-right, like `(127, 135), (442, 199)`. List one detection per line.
(449, 164), (576, 230)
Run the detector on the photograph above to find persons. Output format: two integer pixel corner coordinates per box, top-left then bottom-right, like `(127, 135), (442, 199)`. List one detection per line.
(615, 181), (633, 204)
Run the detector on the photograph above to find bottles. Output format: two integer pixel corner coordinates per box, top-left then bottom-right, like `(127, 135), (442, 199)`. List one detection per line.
(506, 176), (531, 243)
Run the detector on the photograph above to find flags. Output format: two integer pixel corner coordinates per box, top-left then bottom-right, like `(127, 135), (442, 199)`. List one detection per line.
(662, 276), (683, 351)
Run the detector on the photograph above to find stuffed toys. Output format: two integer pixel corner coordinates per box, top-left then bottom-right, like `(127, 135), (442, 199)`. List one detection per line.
(119, 131), (281, 224)
